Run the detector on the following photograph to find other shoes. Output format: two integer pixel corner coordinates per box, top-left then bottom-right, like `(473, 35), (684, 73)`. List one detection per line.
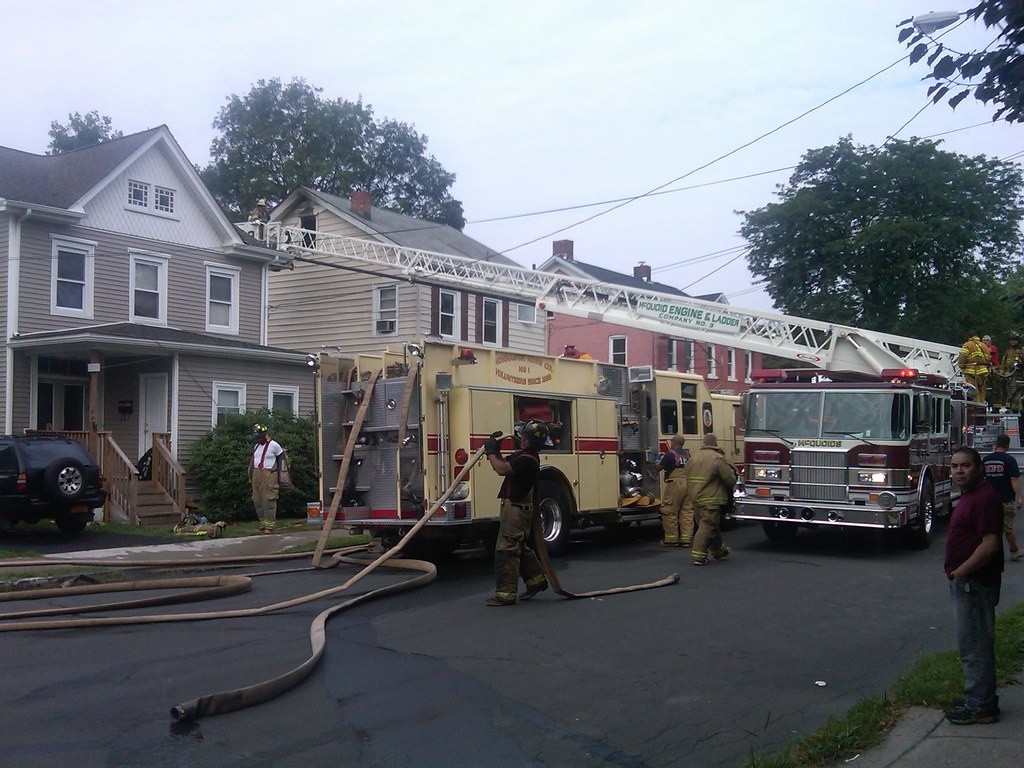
(1010, 547), (1024, 560)
(694, 559), (710, 565)
(714, 547), (730, 559)
(679, 541), (691, 547)
(265, 530), (271, 534)
(519, 579), (548, 600)
(259, 525), (265, 530)
(658, 539), (679, 546)
(485, 597), (509, 606)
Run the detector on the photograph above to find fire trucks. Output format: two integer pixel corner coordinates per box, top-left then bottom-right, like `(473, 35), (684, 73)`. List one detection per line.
(258, 222), (1024, 550)
(305, 333), (744, 559)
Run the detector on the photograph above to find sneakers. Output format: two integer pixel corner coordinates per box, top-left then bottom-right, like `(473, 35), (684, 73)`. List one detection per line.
(951, 695), (1000, 714)
(945, 703), (999, 724)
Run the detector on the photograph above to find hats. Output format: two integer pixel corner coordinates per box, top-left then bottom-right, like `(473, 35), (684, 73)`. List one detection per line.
(670, 435), (686, 446)
(700, 433), (721, 450)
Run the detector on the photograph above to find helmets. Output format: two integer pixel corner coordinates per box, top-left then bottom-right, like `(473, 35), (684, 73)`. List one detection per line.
(257, 199), (266, 206)
(183, 514), (201, 526)
(252, 422), (268, 437)
(1007, 331), (1020, 340)
(515, 419), (554, 447)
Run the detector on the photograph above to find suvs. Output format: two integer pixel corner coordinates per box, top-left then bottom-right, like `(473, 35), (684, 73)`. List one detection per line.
(0, 434), (107, 534)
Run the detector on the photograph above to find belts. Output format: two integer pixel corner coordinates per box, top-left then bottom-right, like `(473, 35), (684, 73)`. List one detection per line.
(501, 502), (531, 510)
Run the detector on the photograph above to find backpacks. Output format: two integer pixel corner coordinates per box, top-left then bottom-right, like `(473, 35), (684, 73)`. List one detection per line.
(130, 447), (154, 481)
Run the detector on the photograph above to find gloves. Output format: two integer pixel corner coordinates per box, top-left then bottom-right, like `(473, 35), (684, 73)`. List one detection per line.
(489, 430), (503, 452)
(484, 439), (500, 460)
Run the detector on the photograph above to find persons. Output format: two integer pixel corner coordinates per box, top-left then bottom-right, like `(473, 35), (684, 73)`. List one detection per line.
(484, 420), (549, 606)
(251, 423), (285, 533)
(1001, 331), (1024, 412)
(685, 434), (736, 565)
(945, 447), (1004, 724)
(983, 434), (1024, 560)
(654, 435), (694, 546)
(959, 330), (1003, 404)
